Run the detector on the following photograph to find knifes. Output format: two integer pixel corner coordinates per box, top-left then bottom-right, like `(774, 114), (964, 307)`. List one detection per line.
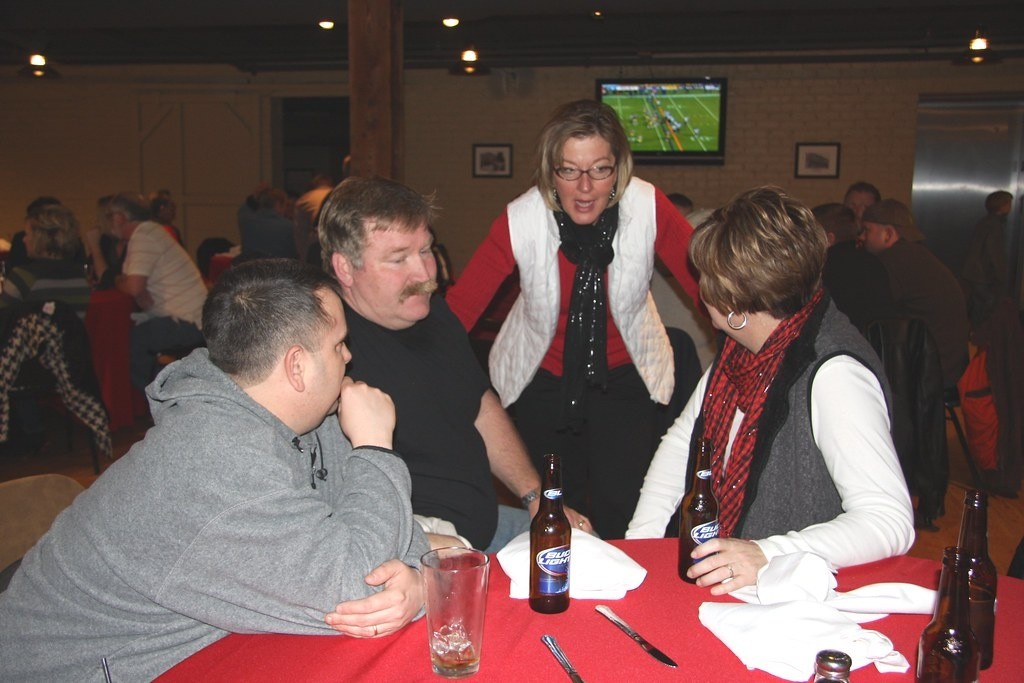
(595, 605), (680, 668)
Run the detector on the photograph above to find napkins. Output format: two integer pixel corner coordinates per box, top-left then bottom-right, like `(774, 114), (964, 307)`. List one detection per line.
(497, 526), (648, 599)
(698, 549), (940, 681)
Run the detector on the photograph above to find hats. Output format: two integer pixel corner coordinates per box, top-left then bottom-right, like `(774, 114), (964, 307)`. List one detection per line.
(862, 198), (926, 241)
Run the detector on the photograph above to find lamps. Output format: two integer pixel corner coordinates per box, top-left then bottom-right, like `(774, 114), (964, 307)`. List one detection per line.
(448, 50), (493, 78)
(948, 26), (1003, 64)
(17, 54), (65, 81)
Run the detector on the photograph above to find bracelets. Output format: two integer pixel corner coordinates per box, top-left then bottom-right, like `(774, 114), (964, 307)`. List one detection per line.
(521, 482), (540, 508)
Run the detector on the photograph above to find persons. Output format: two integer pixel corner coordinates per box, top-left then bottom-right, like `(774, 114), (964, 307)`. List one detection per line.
(956, 190), (1014, 383)
(237, 171), (328, 262)
(318, 172), (600, 561)
(624, 181), (915, 595)
(1, 257), (439, 683)
(808, 182), (970, 404)
(650, 194), (718, 419)
(1, 187), (235, 478)
(295, 185), (336, 259)
(444, 100), (726, 539)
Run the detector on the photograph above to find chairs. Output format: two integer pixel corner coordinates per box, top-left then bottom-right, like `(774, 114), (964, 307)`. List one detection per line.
(0, 238), (237, 574)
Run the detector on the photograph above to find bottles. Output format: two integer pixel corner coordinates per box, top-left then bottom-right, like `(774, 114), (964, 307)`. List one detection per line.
(677, 437), (720, 584)
(955, 488), (997, 669)
(529, 453), (571, 613)
(813, 650), (852, 683)
(916, 547), (981, 683)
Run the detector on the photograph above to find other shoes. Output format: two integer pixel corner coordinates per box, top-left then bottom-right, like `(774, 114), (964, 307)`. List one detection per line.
(913, 511), (940, 530)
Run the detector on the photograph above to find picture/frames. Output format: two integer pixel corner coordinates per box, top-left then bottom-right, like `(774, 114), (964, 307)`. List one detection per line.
(794, 142), (841, 179)
(472, 143), (513, 178)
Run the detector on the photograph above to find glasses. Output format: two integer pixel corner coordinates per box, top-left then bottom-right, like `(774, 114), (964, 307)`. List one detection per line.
(550, 161), (615, 181)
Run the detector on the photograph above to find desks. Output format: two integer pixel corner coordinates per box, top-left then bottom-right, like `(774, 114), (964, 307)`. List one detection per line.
(147, 539), (1024, 683)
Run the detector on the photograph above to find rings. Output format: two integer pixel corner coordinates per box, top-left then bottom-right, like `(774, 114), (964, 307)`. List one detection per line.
(724, 566), (734, 578)
(578, 519), (586, 527)
(372, 626), (379, 636)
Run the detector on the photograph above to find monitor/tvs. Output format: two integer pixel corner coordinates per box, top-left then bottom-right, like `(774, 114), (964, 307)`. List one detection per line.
(595, 78), (726, 166)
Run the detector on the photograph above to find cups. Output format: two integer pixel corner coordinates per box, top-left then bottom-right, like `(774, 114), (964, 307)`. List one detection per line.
(420, 546), (490, 678)
(83, 264), (92, 283)
